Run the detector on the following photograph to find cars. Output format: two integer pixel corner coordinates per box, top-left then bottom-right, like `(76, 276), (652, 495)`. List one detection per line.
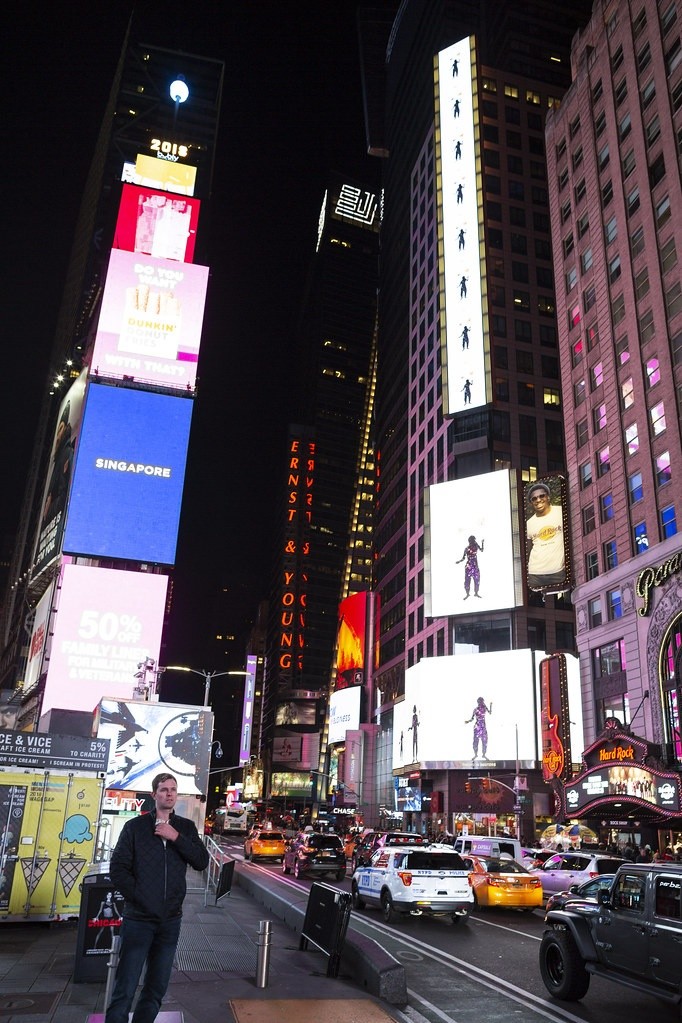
(284, 812), (366, 880)
(242, 814), (289, 861)
(348, 829), (555, 927)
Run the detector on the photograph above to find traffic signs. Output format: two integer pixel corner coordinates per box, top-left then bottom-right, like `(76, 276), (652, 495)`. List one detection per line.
(515, 810), (526, 816)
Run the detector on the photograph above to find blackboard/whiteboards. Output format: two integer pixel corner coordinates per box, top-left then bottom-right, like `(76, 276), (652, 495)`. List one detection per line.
(74, 873), (127, 982)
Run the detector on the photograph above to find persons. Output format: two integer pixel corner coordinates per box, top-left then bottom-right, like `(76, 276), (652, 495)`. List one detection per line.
(568, 842), (577, 852)
(556, 843), (564, 852)
(105, 773), (210, 1023)
(504, 832), (542, 851)
(422, 832), (455, 845)
(608, 776), (653, 799)
(42, 399), (78, 521)
(92, 892), (123, 949)
(0, 706), (20, 729)
(598, 842), (682, 863)
(301, 821), (361, 838)
(526, 483), (565, 588)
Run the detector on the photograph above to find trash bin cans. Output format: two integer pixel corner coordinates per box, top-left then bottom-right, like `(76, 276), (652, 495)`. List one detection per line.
(210, 826), (221, 851)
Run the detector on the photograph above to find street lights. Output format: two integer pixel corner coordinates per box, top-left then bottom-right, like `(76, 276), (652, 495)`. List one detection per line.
(164, 665), (252, 706)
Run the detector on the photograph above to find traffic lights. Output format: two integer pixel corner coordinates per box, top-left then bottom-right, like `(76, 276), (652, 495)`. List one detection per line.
(246, 767), (251, 780)
(214, 784), (221, 793)
(309, 774), (314, 786)
(482, 779), (489, 790)
(464, 781), (470, 794)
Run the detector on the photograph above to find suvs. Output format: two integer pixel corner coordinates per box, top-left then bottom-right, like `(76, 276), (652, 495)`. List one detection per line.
(534, 850), (634, 898)
(538, 862), (682, 999)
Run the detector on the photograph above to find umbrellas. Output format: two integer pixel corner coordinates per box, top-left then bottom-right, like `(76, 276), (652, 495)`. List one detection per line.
(539, 824), (597, 844)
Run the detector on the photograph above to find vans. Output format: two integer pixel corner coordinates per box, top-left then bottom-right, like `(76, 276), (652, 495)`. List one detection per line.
(204, 806), (252, 837)
